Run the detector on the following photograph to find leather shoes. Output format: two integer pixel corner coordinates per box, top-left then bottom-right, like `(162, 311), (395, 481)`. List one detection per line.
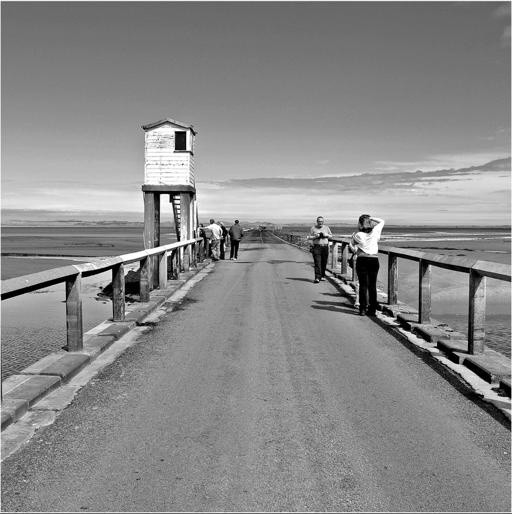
(314, 278), (319, 283)
(320, 276), (325, 281)
(360, 310), (365, 316)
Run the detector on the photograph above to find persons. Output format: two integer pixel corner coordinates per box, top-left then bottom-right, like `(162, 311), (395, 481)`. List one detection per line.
(348, 238), (369, 315)
(199, 218), (244, 261)
(348, 213), (385, 318)
(306, 215), (333, 282)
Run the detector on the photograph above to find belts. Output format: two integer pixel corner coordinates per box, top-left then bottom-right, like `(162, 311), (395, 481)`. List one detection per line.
(319, 244), (327, 247)
